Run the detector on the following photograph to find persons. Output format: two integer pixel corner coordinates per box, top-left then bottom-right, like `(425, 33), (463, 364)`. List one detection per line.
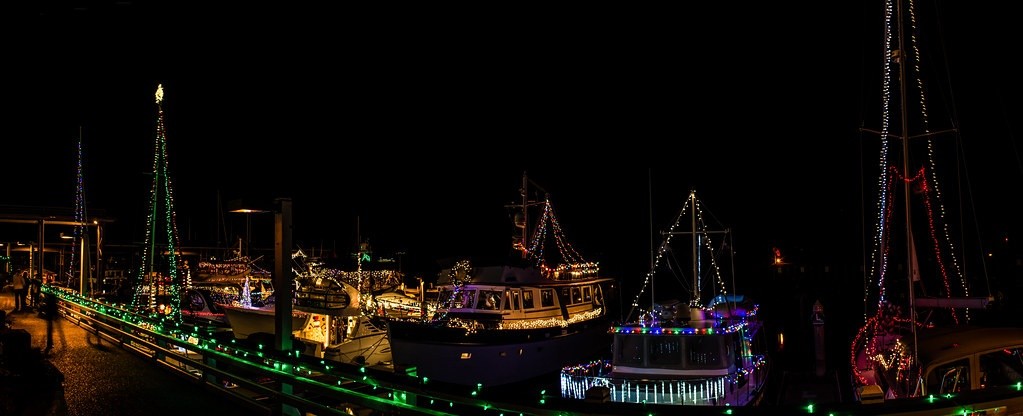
(457, 292), (496, 309)
(11, 270), (53, 314)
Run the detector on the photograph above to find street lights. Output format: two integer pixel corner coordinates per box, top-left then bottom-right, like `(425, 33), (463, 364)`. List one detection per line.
(60, 232), (88, 296)
(0, 242), (10, 273)
(17, 242), (34, 278)
(230, 199), (294, 416)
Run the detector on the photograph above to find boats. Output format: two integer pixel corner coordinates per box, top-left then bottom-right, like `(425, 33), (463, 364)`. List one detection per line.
(57, 127), (122, 301)
(850, 0), (1023, 416)
(561, 190), (771, 416)
(120, 83), (615, 372)
